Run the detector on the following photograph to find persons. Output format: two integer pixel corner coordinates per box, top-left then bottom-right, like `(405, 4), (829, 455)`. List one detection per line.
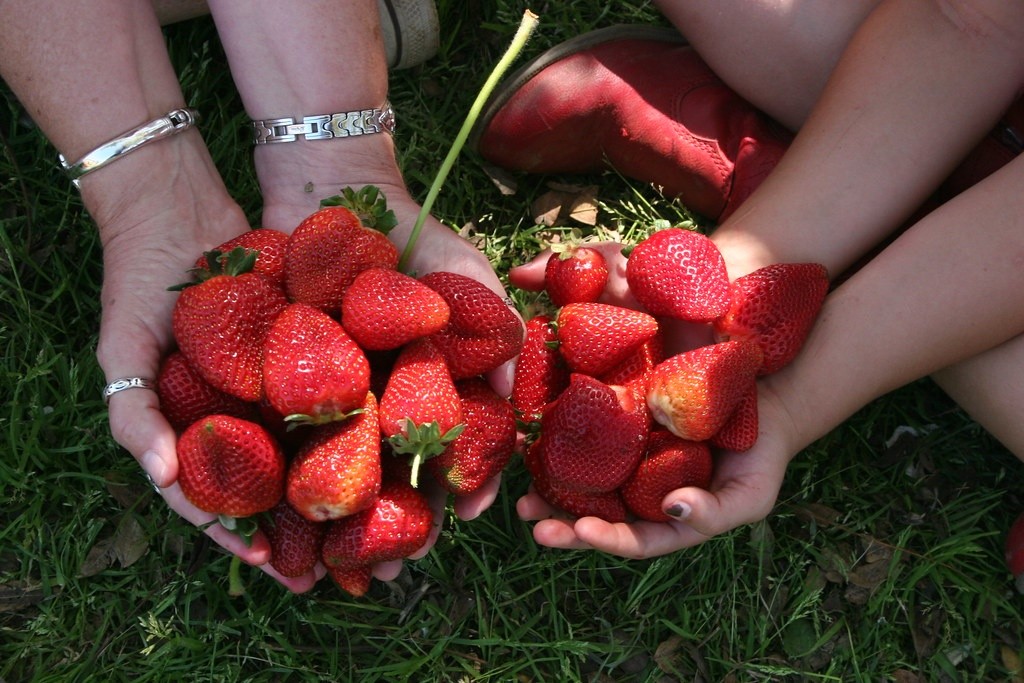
(0, 0), (528, 597)
(473, 0), (1024, 560)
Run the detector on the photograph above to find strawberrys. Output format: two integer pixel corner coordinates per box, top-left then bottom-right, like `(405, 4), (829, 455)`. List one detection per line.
(158, 186), (523, 597)
(513, 219), (828, 522)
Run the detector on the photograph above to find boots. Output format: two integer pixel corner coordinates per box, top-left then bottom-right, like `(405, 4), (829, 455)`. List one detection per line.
(471, 22), (794, 220)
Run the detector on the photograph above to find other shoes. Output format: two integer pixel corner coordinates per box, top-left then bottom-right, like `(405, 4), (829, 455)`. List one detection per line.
(378, 0), (442, 69)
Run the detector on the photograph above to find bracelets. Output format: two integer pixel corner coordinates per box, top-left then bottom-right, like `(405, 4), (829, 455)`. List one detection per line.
(59, 107), (201, 191)
(251, 109), (396, 145)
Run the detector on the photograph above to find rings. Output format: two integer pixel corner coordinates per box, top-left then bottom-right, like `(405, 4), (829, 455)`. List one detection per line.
(103, 377), (154, 406)
